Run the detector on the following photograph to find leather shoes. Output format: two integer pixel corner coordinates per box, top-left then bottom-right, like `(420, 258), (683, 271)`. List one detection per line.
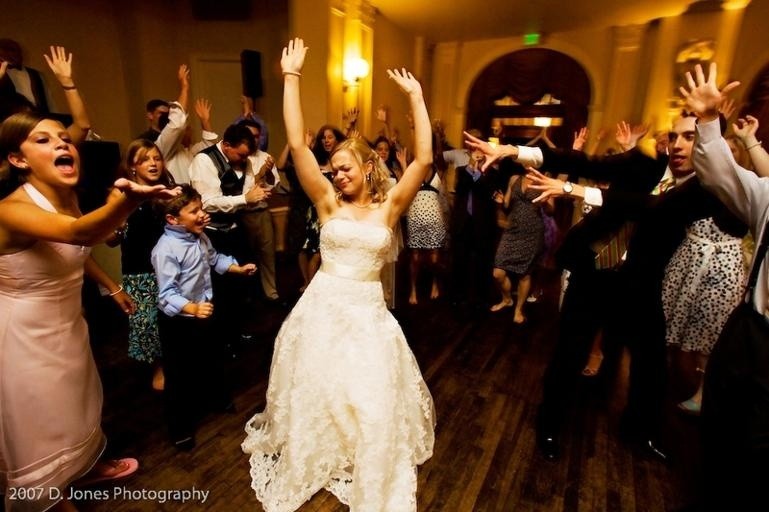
(679, 396), (702, 415)
(543, 435), (557, 461)
(645, 435), (671, 463)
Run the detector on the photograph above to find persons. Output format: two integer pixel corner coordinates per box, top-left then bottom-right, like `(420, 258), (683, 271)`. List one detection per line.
(1, 38), (359, 512)
(368, 63), (768, 511)
(240, 38), (438, 512)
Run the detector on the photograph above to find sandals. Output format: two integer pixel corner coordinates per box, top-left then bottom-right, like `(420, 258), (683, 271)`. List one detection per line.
(582, 353), (603, 376)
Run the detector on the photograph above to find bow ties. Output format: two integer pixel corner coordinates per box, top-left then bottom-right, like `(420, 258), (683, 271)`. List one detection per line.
(7, 64), (23, 71)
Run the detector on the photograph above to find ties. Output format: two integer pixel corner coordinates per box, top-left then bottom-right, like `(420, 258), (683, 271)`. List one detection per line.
(595, 178), (676, 269)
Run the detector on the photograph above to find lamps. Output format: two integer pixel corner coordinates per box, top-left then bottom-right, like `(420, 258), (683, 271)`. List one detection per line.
(344, 57), (370, 82)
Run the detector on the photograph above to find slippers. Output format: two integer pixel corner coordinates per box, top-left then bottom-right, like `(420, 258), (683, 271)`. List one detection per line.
(73, 457), (138, 488)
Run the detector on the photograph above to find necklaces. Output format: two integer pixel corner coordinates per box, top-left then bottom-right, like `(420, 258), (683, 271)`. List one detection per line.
(350, 199), (372, 208)
